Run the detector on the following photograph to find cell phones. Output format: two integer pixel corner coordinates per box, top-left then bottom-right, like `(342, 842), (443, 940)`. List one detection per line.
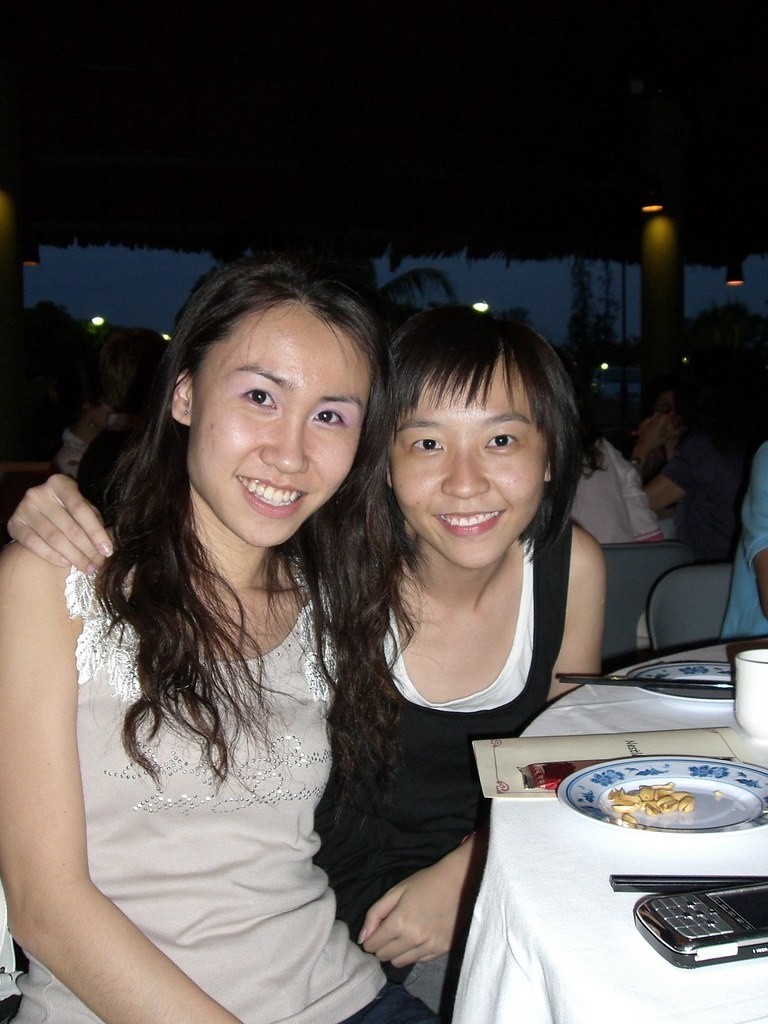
(634, 881), (768, 969)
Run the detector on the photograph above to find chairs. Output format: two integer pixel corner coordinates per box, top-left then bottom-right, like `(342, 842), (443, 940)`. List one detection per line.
(590, 540), (733, 676)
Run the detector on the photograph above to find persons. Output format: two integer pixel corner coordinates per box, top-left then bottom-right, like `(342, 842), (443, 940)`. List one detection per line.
(6, 311), (607, 1011)
(566, 373), (753, 565)
(0, 258), (440, 1024)
(44, 327), (166, 521)
(720, 442), (768, 640)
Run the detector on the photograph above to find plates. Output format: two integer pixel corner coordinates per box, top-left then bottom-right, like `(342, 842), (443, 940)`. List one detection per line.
(557, 755), (768, 834)
(627, 661), (735, 704)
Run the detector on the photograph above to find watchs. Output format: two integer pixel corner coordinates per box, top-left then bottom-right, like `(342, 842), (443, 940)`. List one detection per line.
(628, 457), (649, 470)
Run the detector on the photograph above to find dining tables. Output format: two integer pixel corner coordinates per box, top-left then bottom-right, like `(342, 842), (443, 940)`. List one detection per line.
(448, 638), (767, 1024)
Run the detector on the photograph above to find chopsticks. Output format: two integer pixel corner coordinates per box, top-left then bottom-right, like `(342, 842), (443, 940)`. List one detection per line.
(555, 673), (735, 690)
(609, 875), (768, 893)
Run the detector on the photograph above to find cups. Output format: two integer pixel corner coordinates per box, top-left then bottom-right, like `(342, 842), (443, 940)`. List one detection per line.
(735, 649), (768, 745)
